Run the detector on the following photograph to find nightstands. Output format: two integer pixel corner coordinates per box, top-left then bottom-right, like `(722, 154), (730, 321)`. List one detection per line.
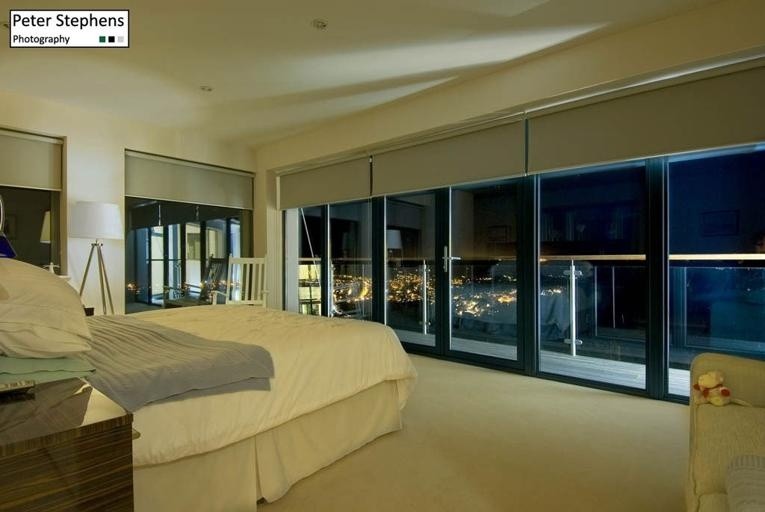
(1, 376), (134, 512)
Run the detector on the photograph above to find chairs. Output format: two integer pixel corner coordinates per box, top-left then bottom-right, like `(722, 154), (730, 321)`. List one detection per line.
(211, 253), (269, 307)
(685, 352), (765, 511)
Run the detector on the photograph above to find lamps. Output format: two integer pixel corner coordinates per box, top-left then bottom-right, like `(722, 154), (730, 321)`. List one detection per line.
(69, 201), (126, 315)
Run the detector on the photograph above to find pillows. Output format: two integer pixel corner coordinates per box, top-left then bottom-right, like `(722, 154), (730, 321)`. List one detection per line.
(0, 256), (95, 390)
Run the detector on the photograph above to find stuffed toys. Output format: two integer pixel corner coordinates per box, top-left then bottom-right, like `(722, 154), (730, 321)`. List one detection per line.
(696, 369), (733, 407)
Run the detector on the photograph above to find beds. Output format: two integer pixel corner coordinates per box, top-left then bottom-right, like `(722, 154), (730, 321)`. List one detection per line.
(1, 303), (404, 511)
